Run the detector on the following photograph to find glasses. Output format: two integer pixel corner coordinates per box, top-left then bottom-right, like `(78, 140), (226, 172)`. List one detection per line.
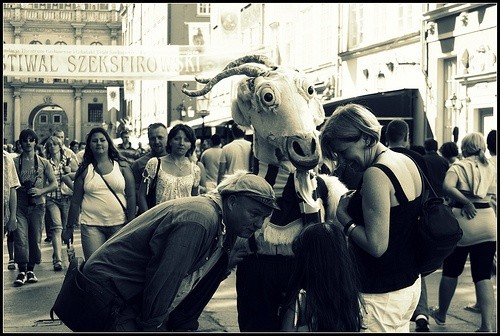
(23, 138), (35, 142)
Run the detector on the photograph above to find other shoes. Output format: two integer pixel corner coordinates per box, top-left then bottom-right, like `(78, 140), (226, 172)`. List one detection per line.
(415, 315), (430, 331)
(8, 260), (15, 269)
(464, 304), (480, 312)
(53, 254), (63, 270)
(45, 236), (52, 242)
(14, 273), (27, 285)
(27, 271), (38, 282)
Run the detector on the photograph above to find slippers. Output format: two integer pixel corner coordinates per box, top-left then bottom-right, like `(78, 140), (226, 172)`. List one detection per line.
(429, 306), (446, 325)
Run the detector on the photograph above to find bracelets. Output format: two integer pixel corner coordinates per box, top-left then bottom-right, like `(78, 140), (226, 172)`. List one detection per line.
(344, 219), (358, 236)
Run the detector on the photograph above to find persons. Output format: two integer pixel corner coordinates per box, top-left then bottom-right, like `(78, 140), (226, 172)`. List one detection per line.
(11, 129), (58, 285)
(51, 173), (276, 332)
(4, 149), (22, 236)
(181, 55), (329, 332)
(137, 124), (201, 215)
(116, 131), (146, 169)
(43, 136), (79, 272)
(194, 123), (259, 194)
(131, 122), (169, 191)
(63, 128), (137, 263)
(319, 103), (422, 332)
(382, 119), (497, 333)
(3, 138), (87, 243)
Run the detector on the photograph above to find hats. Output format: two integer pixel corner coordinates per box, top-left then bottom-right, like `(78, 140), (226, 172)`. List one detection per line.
(218, 173), (280, 210)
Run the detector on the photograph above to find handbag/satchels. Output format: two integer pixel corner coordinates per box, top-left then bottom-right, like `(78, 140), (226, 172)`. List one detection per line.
(136, 156), (161, 217)
(61, 158), (74, 197)
(402, 151), (463, 273)
(51, 257), (117, 332)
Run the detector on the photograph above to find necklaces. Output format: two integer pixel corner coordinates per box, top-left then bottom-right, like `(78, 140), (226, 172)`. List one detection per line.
(174, 162), (186, 171)
